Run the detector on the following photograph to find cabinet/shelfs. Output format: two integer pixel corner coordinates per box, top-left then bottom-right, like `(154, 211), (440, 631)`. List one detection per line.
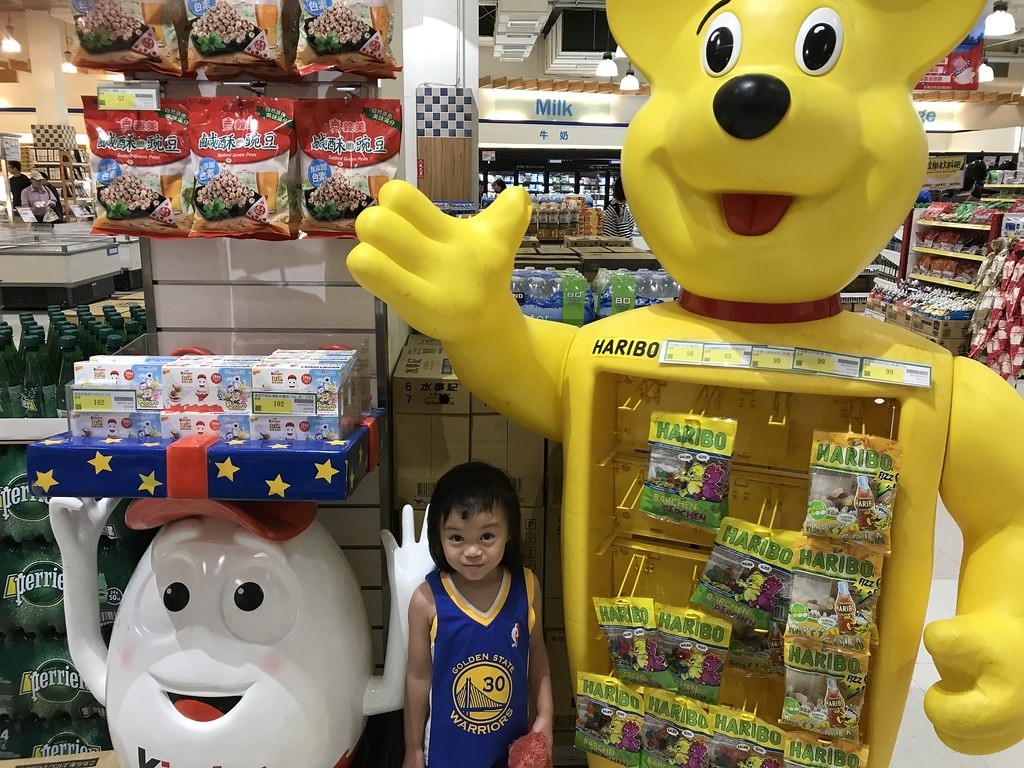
(842, 181), (1024, 324)
(480, 171), (624, 221)
(0, 148), (98, 223)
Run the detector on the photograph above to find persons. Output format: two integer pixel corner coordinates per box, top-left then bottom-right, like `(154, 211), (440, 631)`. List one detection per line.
(7, 161), (64, 225)
(494, 178), (506, 194)
(402, 461), (553, 768)
(479, 179), (484, 205)
(601, 176), (634, 243)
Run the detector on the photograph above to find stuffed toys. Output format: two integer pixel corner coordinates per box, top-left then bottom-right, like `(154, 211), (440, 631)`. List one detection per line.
(508, 719), (551, 768)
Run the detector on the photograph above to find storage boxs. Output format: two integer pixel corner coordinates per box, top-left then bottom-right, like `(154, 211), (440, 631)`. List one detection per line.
(71, 348), (372, 442)
(514, 199), (662, 284)
(842, 278), (973, 356)
(390, 333), (583, 744)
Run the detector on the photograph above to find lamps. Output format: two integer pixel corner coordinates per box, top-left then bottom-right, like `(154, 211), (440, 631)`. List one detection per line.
(619, 60), (639, 90)
(983, 0), (1016, 36)
(2, 11), (20, 53)
(978, 44), (994, 82)
(62, 20), (77, 74)
(596, 30), (619, 77)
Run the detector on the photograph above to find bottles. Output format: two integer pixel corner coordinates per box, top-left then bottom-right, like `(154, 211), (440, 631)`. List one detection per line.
(481, 193), (500, 209)
(512, 265), (679, 328)
(861, 269), (878, 275)
(882, 237), (902, 251)
(877, 255), (900, 282)
(0, 305), (146, 418)
(840, 297), (866, 304)
(432, 200), (476, 217)
(526, 193), (593, 233)
